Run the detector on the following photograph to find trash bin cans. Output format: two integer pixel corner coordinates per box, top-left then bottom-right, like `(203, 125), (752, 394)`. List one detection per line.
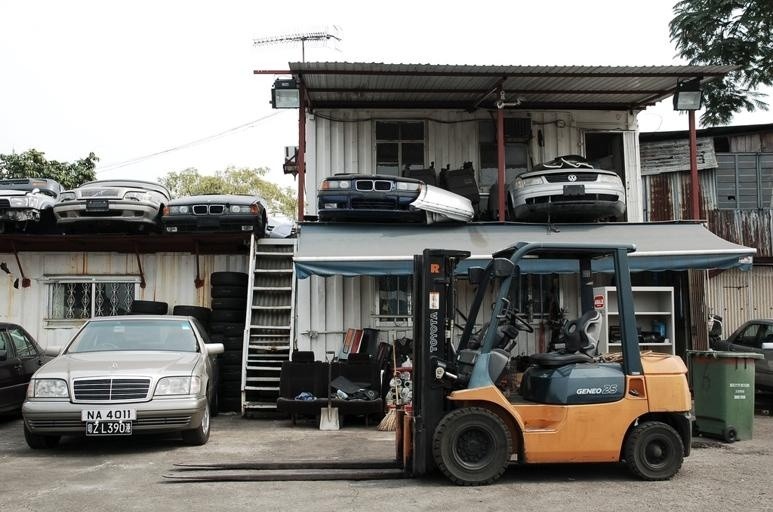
(686, 349), (765, 444)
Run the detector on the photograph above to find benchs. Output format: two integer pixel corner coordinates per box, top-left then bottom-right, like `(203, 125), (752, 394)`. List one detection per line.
(277, 361), (382, 427)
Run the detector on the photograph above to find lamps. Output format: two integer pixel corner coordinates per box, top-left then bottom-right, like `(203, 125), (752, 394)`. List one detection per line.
(673, 78), (703, 110)
(269, 76), (301, 109)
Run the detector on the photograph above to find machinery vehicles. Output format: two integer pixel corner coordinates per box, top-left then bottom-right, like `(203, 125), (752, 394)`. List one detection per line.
(156, 240), (700, 490)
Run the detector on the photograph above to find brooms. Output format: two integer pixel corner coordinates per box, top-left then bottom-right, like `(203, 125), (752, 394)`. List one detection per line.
(376, 334), (399, 431)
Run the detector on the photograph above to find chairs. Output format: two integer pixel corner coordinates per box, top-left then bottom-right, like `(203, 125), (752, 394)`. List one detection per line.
(522, 310), (603, 401)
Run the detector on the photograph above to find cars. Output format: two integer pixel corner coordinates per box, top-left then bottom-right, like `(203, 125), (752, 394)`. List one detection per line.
(713, 316), (772, 392)
(0, 178), (64, 235)
(23, 312), (225, 447)
(52, 178), (171, 236)
(0, 320), (55, 424)
(160, 192), (266, 237)
(312, 168), (428, 220)
(489, 153), (627, 223)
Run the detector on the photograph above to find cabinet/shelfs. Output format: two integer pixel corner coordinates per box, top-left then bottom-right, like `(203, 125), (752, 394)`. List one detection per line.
(593, 286), (675, 355)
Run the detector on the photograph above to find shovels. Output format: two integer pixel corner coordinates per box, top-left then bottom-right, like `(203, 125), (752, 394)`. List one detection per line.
(319, 351), (340, 431)
